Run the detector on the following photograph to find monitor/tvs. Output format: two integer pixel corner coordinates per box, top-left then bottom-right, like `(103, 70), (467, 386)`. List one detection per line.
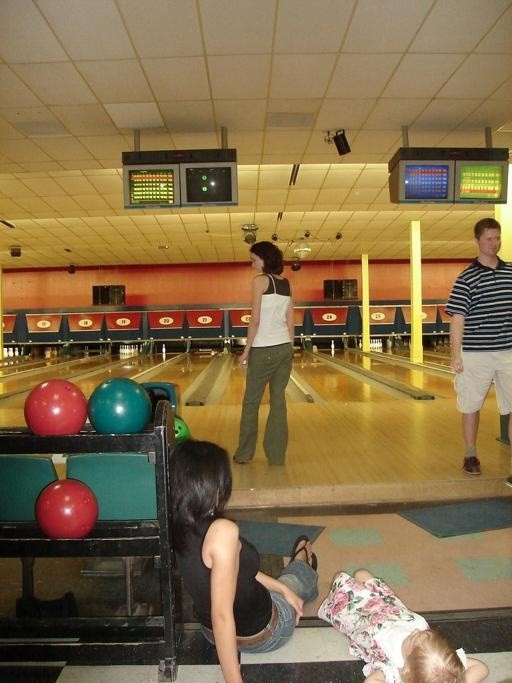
(123, 164), (180, 208)
(180, 162), (237, 207)
(389, 159), (455, 203)
(455, 161), (508, 204)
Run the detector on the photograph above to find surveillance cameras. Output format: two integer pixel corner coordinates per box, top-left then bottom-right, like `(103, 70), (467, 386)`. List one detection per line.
(333, 134), (350, 155)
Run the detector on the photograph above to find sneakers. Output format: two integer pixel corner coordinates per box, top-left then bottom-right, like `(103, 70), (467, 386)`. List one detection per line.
(462, 455), (483, 474)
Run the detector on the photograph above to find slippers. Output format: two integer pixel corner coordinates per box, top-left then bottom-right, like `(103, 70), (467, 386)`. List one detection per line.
(288, 536), (318, 572)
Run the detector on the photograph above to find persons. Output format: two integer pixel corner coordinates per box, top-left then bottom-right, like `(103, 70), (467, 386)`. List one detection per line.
(170, 437), (319, 683)
(232, 241), (296, 465)
(444, 217), (512, 476)
(318, 570), (490, 683)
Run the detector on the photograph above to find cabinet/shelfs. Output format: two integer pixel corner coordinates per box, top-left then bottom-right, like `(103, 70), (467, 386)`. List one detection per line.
(0, 400), (180, 683)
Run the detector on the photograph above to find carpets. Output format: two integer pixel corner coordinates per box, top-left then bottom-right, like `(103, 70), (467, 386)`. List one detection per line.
(233, 519), (325, 557)
(397, 498), (512, 538)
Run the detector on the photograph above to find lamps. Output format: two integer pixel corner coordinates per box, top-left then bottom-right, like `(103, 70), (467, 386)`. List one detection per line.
(324, 129), (351, 156)
